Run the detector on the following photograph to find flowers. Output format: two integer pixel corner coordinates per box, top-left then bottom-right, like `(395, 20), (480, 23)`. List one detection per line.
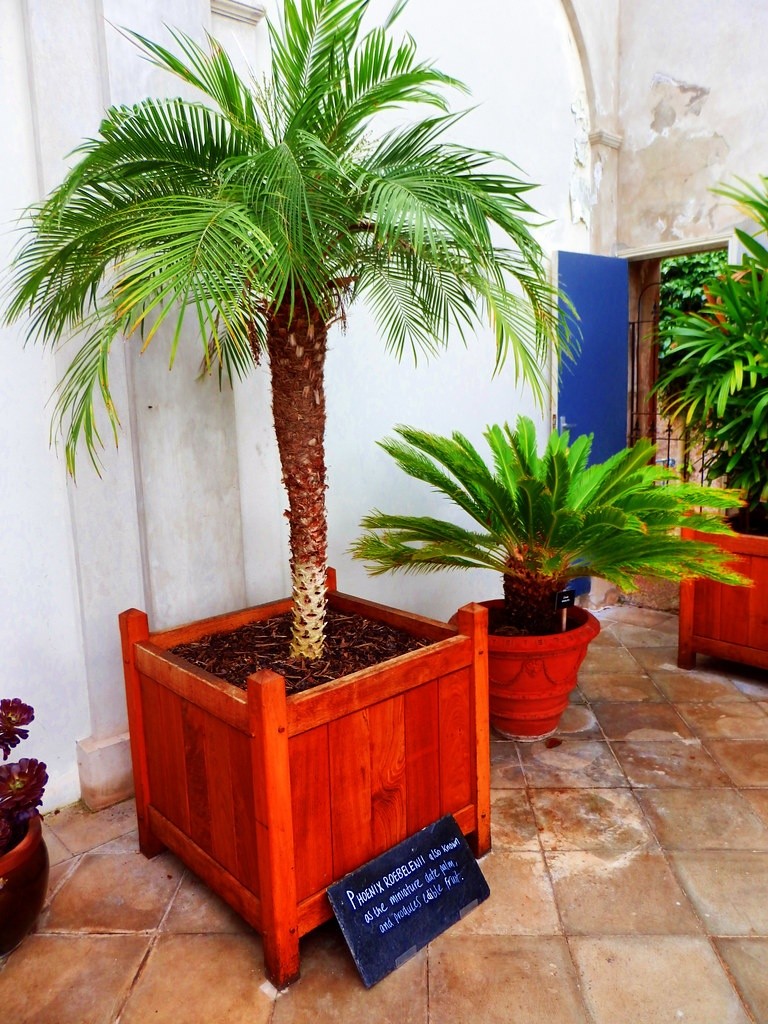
(0, 697), (49, 821)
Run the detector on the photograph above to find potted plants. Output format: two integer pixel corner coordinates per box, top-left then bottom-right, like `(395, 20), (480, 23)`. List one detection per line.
(344, 413), (753, 742)
(641, 173), (768, 676)
(1, 0), (586, 993)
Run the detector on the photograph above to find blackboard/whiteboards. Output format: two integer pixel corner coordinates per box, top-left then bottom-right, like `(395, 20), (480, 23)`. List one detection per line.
(326, 812), (490, 990)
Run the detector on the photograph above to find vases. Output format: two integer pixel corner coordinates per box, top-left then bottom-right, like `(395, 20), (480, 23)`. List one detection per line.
(0, 813), (50, 958)
